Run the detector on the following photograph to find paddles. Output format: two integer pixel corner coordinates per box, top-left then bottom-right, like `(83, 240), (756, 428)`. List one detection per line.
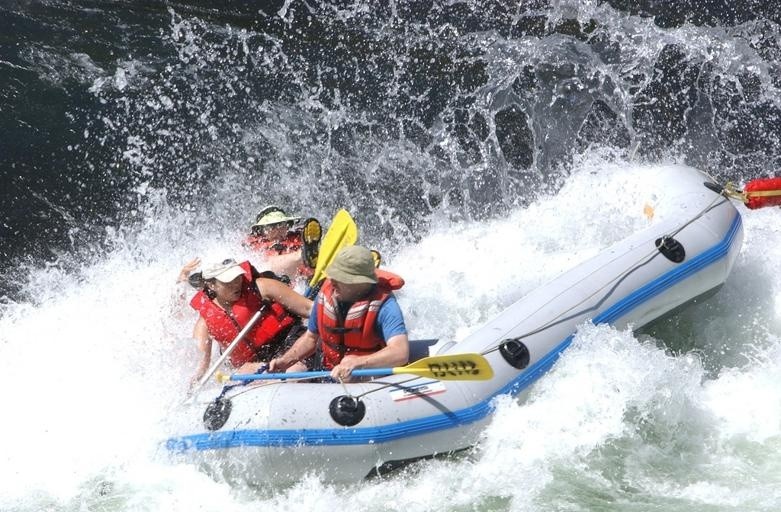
(301, 205), (358, 297)
(218, 355), (492, 384)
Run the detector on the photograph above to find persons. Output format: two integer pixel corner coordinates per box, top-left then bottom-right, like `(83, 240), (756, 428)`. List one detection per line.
(266, 245), (410, 381)
(164, 216), (322, 319)
(183, 251), (315, 395)
(240, 208), (324, 289)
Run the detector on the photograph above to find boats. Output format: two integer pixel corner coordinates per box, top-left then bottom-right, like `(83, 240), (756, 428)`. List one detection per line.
(163, 162), (745, 487)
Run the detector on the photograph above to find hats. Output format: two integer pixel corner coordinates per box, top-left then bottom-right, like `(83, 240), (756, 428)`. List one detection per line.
(326, 246), (378, 285)
(250, 206), (302, 234)
(187, 250), (247, 288)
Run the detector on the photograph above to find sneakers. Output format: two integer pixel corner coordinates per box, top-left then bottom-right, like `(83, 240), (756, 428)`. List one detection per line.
(301, 217), (322, 268)
